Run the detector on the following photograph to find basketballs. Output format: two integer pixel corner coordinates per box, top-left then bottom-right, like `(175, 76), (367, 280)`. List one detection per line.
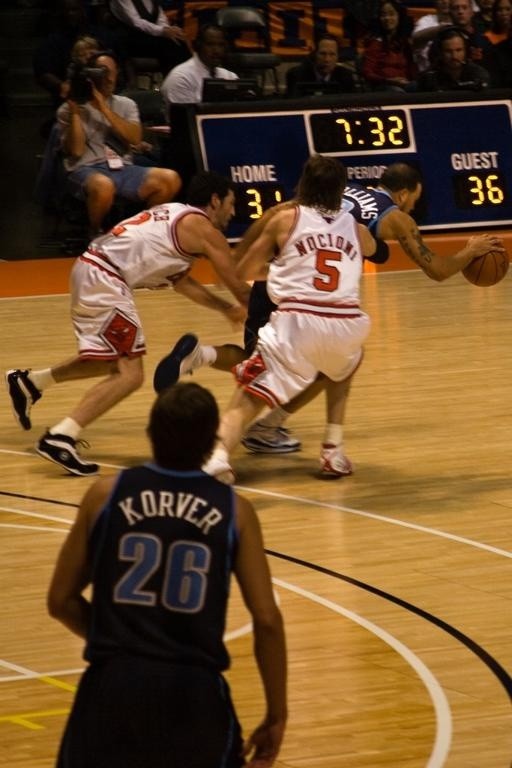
(461, 239), (510, 287)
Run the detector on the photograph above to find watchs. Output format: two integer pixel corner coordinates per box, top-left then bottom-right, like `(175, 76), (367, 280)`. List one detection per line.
(67, 109), (80, 116)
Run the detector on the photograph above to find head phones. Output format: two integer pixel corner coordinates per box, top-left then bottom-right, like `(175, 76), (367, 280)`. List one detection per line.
(427, 25), (473, 64)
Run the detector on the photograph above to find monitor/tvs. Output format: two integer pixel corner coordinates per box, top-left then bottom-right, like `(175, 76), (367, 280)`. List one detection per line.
(200, 77), (258, 114)
(294, 81), (338, 98)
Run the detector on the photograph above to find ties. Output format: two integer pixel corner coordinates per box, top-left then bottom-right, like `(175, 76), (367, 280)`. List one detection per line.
(209, 69), (217, 78)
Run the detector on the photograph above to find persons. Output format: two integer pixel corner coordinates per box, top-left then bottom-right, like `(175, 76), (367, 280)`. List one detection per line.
(202, 156), (389, 484)
(160, 26), (240, 124)
(101, 0), (189, 54)
(285, 34), (354, 97)
(48, 382), (286, 768)
(473, 0), (496, 30)
(449, 0), (492, 68)
(153, 164), (506, 454)
(129, 140), (162, 183)
(5, 172), (252, 476)
(411, 27), (491, 92)
(57, 54), (182, 240)
(362, 0), (420, 86)
(60, 37), (107, 100)
(412, 0), (454, 72)
(483, 0), (512, 86)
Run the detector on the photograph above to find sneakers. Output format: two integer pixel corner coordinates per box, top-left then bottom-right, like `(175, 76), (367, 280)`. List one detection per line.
(153, 334), (203, 397)
(317, 448), (352, 477)
(6, 369), (42, 431)
(36, 427), (100, 476)
(202, 459), (236, 486)
(240, 425), (302, 454)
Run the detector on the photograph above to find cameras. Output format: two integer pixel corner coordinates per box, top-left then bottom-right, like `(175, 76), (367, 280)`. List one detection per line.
(71, 65), (109, 105)
